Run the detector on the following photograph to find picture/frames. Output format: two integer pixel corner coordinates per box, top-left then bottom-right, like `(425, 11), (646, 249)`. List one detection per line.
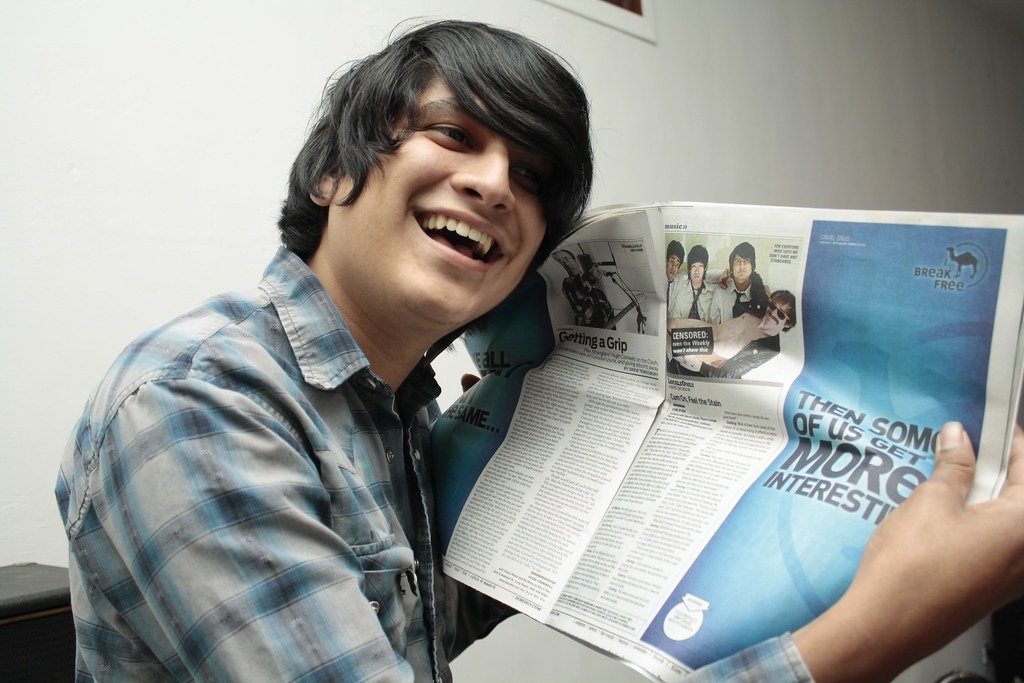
(542, 0), (654, 42)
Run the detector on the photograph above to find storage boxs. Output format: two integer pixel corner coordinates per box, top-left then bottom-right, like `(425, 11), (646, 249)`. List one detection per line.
(0, 564), (77, 683)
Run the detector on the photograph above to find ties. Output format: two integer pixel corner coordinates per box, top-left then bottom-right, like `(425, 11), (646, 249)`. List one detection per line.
(734, 291), (743, 317)
(688, 288), (701, 320)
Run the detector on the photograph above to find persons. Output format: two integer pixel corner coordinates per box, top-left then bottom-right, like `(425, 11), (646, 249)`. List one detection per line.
(52, 17), (1023, 683)
(664, 238), (797, 381)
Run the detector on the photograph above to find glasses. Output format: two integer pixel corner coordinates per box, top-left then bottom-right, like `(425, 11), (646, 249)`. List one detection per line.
(768, 301), (789, 320)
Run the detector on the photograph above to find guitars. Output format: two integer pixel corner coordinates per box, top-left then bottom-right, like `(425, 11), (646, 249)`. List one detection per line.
(577, 294), (645, 330)
